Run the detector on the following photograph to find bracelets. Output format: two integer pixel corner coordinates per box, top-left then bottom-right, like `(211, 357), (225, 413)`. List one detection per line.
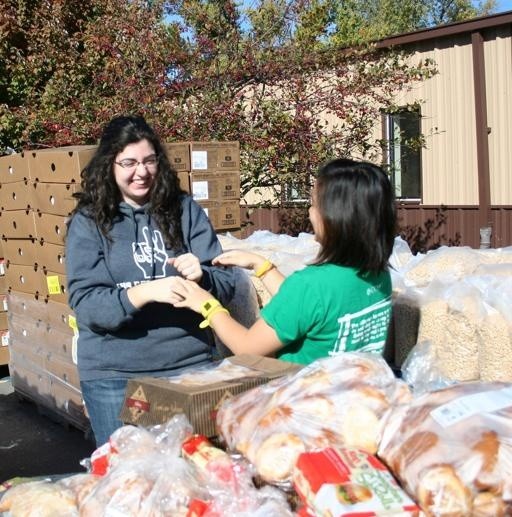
(199, 308), (229, 330)
(254, 260), (271, 278)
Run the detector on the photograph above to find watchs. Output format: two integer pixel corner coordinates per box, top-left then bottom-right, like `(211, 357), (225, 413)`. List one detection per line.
(201, 298), (221, 318)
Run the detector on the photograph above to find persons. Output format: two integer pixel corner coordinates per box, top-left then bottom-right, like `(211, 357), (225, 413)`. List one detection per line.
(172, 159), (397, 367)
(62, 115), (238, 451)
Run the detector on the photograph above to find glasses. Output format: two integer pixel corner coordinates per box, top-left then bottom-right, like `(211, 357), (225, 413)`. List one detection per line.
(113, 156), (161, 169)
